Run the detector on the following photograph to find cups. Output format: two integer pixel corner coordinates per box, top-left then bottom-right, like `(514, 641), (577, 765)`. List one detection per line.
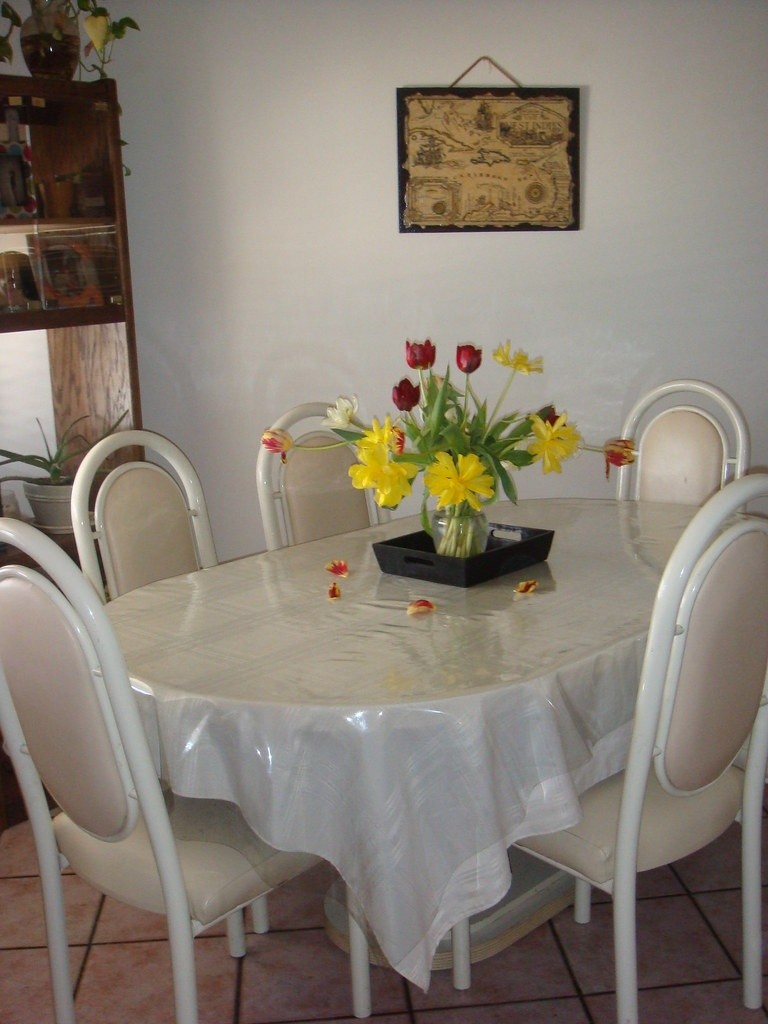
(39, 182), (73, 218)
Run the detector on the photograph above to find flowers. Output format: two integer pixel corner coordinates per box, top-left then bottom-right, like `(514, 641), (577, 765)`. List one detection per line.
(266, 337), (638, 498)
(0, 0), (141, 80)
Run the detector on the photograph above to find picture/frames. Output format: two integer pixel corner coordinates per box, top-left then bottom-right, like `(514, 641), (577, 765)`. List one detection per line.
(395, 85), (581, 232)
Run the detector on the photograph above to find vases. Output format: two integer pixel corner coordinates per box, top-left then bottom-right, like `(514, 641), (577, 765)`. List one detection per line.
(432, 512), (488, 557)
(20, 0), (81, 81)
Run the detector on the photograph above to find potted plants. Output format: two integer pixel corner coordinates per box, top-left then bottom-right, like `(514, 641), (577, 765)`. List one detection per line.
(0, 409), (129, 534)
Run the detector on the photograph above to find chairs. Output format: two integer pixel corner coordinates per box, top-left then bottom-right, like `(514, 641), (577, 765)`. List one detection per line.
(69, 429), (220, 606)
(256, 402), (389, 552)
(449, 473), (768, 1024)
(616, 379), (750, 503)
(0, 513), (374, 1024)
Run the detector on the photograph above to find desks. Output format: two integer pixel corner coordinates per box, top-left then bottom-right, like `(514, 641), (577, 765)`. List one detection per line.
(87, 501), (763, 976)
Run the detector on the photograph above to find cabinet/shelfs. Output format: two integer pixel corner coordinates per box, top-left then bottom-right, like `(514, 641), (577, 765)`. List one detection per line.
(0, 72), (149, 574)
